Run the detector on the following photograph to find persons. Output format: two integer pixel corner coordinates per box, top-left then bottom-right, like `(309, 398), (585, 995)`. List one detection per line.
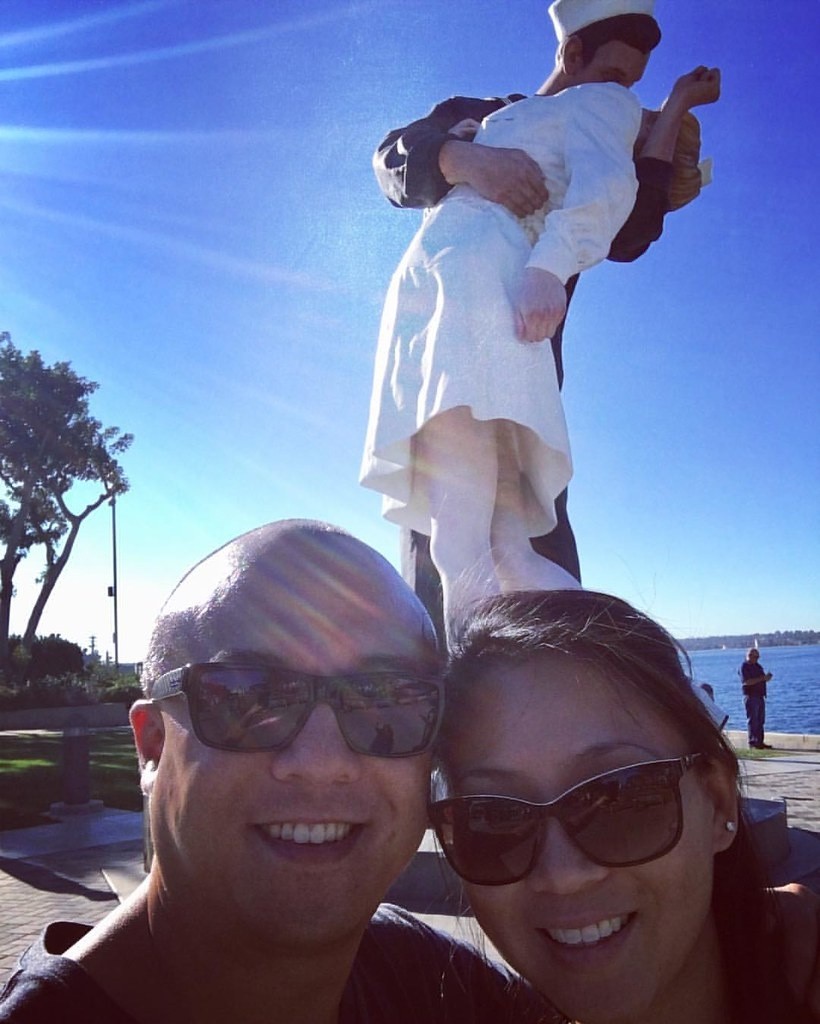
(358, 85), (701, 659)
(371, 0), (720, 631)
(426, 590), (820, 1024)
(738, 648), (774, 750)
(0, 518), (579, 1024)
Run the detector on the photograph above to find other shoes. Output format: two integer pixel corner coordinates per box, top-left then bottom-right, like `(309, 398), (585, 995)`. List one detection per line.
(761, 742), (771, 749)
(749, 743), (764, 750)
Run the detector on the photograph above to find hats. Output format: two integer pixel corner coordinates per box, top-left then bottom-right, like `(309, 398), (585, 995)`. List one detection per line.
(547, 0), (652, 42)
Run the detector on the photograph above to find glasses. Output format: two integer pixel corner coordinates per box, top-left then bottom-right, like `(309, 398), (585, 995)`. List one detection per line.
(427, 752), (708, 885)
(149, 661), (451, 759)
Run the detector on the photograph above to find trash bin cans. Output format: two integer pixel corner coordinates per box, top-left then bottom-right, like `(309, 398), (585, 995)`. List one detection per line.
(63, 714), (90, 804)
